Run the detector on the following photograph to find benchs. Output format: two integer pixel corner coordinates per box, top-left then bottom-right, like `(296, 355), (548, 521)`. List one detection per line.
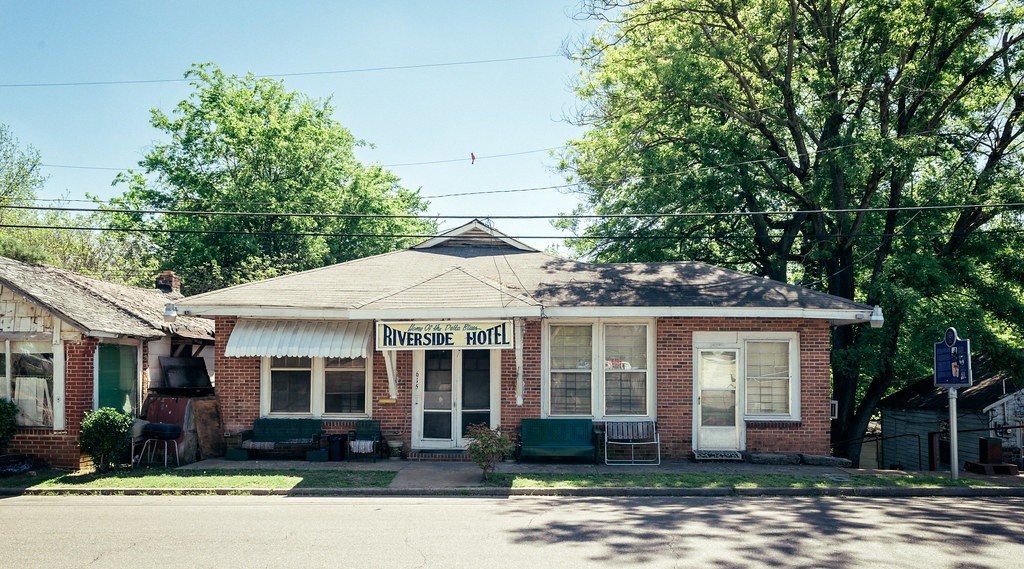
(510, 418), (601, 464)
(604, 420), (661, 466)
(238, 418), (323, 451)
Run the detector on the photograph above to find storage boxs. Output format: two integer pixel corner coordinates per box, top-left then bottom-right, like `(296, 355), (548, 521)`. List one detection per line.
(141, 423), (182, 440)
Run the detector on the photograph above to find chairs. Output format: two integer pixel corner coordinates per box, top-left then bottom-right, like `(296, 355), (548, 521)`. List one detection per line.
(347, 420), (383, 464)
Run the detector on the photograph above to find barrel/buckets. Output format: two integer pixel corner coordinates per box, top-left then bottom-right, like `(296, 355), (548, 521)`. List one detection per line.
(386, 441), (404, 460)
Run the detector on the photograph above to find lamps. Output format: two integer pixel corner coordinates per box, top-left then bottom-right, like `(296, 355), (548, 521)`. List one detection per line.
(162, 303), (178, 324)
(869, 305), (884, 329)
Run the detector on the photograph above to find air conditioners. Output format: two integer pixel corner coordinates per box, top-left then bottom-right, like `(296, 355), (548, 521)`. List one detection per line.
(831, 400), (838, 420)
